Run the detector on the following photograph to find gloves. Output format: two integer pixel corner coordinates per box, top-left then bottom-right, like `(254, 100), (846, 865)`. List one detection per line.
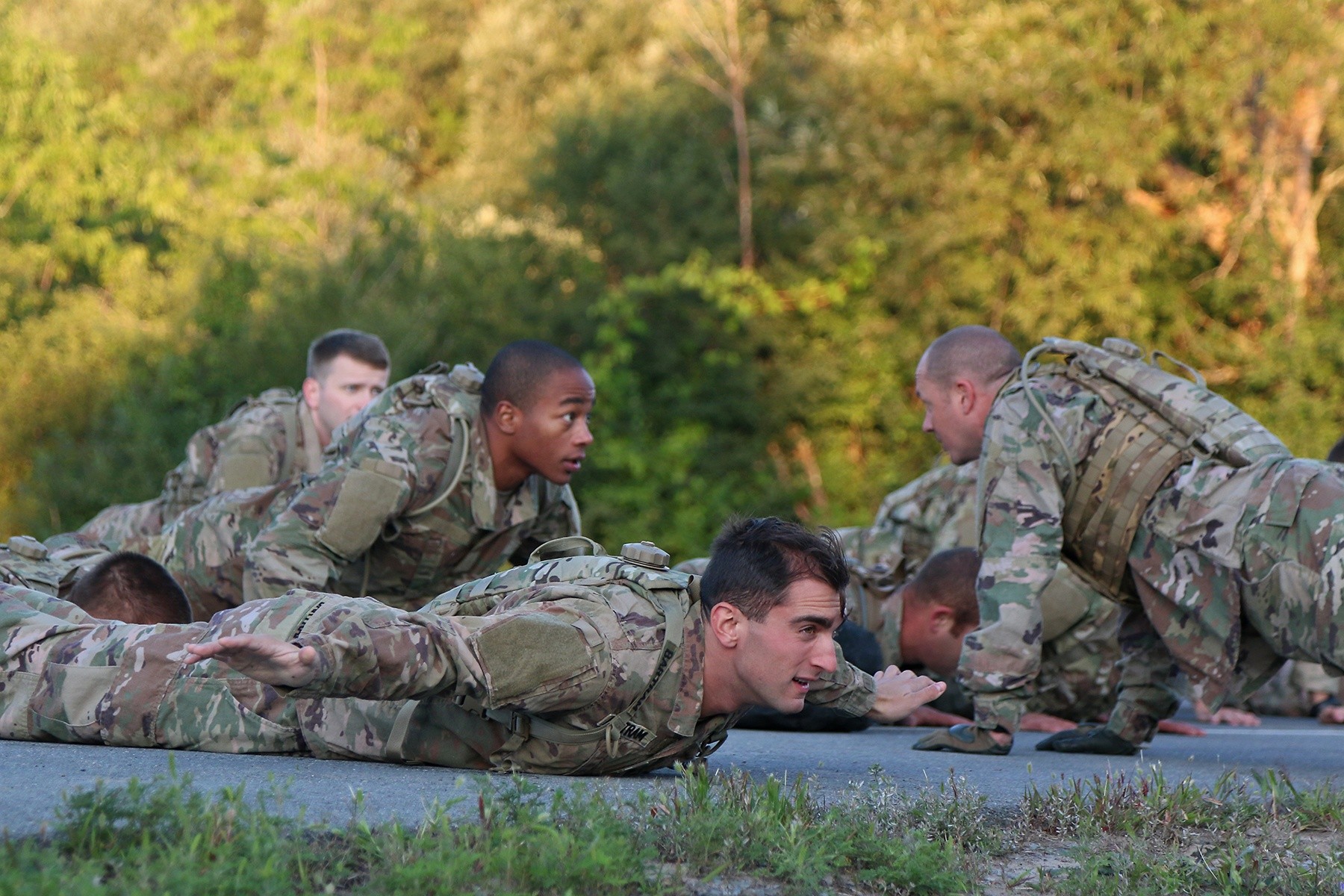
(911, 724), (1015, 754)
(1035, 726), (1138, 757)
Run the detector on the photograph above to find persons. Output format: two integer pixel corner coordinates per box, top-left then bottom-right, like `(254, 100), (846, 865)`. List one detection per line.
(1, 328), (1343, 738)
(0, 514), (946, 777)
(118, 339), (597, 616)
(911, 324), (1343, 756)
